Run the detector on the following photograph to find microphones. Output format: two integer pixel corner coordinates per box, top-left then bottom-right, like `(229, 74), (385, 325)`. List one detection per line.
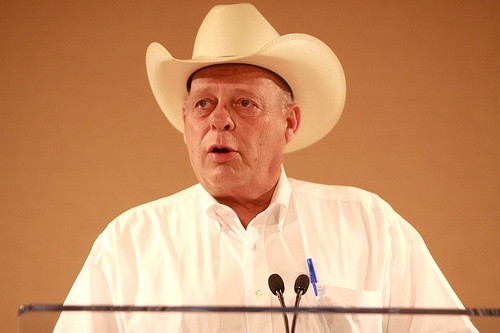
(291, 274), (309, 333)
(268, 274), (289, 333)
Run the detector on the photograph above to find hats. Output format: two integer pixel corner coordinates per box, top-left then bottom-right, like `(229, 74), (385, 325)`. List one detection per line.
(145, 3), (346, 154)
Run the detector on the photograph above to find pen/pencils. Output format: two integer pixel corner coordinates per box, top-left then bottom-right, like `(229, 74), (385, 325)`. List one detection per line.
(307, 258), (318, 296)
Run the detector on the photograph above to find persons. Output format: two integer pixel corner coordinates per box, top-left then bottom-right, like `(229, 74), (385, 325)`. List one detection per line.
(52, 3), (480, 333)
